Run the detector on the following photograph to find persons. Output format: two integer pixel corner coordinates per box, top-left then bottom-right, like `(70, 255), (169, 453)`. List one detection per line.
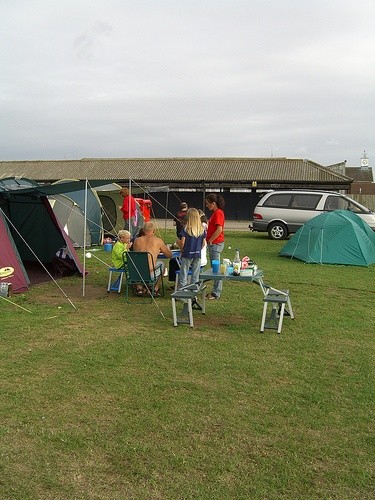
(133, 222), (173, 297)
(111, 227), (145, 279)
(173, 203), (208, 267)
(176, 207), (206, 290)
(119, 188), (136, 230)
(205, 194), (227, 300)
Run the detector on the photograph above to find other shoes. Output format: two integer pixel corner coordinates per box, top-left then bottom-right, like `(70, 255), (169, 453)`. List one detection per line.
(136, 288), (148, 295)
(205, 293), (219, 299)
(167, 286), (175, 292)
(151, 290), (162, 297)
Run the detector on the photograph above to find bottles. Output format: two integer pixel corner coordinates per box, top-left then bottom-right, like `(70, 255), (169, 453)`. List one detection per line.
(235, 249), (239, 259)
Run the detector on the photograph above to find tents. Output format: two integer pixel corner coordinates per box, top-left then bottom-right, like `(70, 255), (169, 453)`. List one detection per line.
(277, 209), (375, 268)
(0, 179), (140, 295)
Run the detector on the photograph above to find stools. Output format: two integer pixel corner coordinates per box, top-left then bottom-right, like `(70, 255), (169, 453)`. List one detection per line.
(170, 291), (198, 329)
(108, 267), (126, 294)
(260, 296), (289, 334)
(268, 289), (294, 320)
(183, 284), (207, 316)
(175, 265), (206, 293)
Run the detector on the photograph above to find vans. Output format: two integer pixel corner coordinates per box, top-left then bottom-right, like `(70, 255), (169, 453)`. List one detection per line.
(249, 189), (375, 240)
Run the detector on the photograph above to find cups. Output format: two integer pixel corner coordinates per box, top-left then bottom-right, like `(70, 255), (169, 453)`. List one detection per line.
(233, 262), (240, 273)
(212, 260), (220, 273)
(221, 259), (229, 275)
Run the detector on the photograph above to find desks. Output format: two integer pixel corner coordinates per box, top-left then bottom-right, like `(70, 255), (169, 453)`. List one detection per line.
(157, 249), (182, 271)
(171, 265), (294, 335)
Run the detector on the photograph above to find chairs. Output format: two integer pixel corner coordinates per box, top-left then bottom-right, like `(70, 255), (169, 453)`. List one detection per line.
(121, 250), (165, 305)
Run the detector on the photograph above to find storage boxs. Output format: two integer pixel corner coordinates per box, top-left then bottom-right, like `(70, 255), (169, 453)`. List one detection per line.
(0, 282), (13, 297)
